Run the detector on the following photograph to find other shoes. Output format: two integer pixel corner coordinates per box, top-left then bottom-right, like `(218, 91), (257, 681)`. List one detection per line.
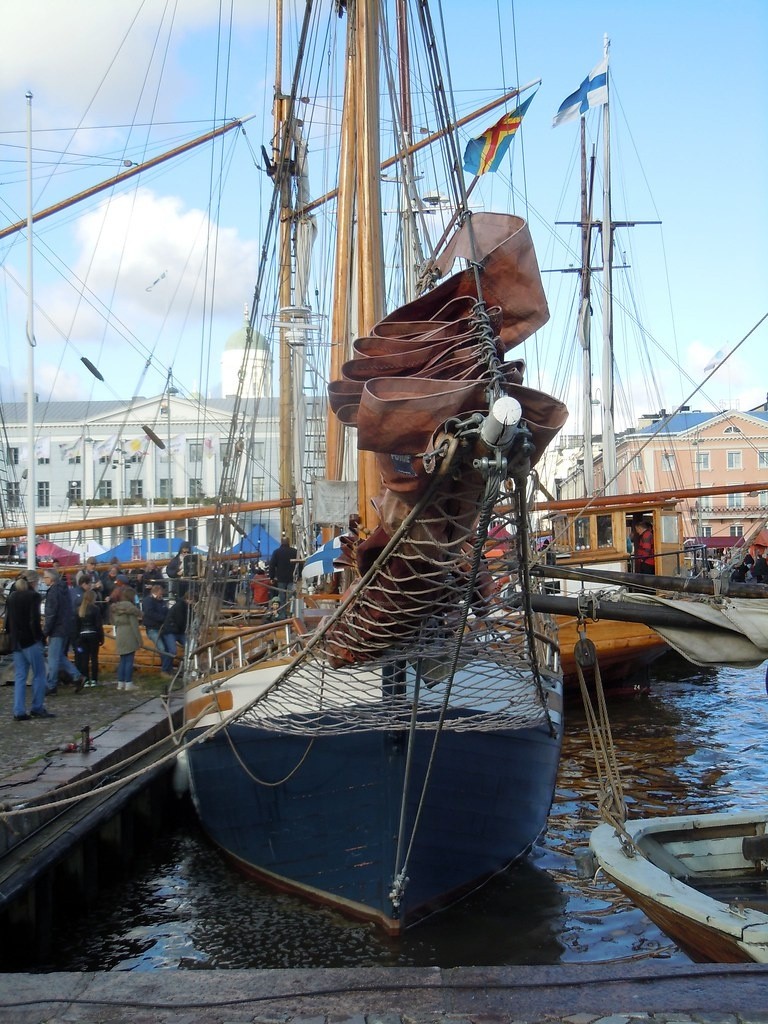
(14, 714), (32, 720)
(117, 681), (125, 689)
(125, 681), (140, 690)
(90, 680), (101, 687)
(74, 674), (88, 694)
(160, 672), (170, 677)
(84, 680), (90, 687)
(45, 686), (57, 696)
(30, 707), (56, 718)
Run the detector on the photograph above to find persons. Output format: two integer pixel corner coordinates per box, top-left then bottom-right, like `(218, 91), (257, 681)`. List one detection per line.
(537, 539), (550, 551)
(59, 552), (274, 620)
(109, 586), (144, 690)
(634, 519), (655, 574)
(161, 590), (198, 677)
(74, 590), (105, 688)
(251, 568), (274, 607)
(269, 536), (303, 616)
(3, 570), (57, 720)
(142, 584), (177, 677)
(262, 596), (287, 623)
(43, 567), (89, 695)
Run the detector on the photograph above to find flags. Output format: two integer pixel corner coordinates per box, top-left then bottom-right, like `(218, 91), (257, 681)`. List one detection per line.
(300, 533), (356, 578)
(462, 84), (542, 177)
(551, 38), (610, 128)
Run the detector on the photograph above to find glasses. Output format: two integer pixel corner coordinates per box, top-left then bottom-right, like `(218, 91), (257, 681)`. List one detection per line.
(182, 551), (189, 554)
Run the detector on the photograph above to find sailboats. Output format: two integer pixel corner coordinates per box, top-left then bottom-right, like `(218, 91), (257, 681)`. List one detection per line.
(0, 0), (767, 937)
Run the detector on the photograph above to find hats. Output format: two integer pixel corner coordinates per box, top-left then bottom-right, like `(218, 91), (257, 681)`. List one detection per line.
(87, 557), (98, 564)
(272, 597), (280, 604)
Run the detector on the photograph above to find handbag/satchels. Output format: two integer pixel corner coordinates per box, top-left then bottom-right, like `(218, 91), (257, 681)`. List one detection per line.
(0, 591), (15, 654)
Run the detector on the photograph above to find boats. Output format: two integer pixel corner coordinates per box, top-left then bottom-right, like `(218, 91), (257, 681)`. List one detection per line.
(575, 808), (768, 966)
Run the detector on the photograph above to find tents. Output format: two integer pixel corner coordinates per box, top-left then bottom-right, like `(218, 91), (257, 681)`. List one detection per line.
(91, 536), (210, 579)
(480, 524), (515, 549)
(6, 534), (81, 590)
(221, 523), (281, 575)
